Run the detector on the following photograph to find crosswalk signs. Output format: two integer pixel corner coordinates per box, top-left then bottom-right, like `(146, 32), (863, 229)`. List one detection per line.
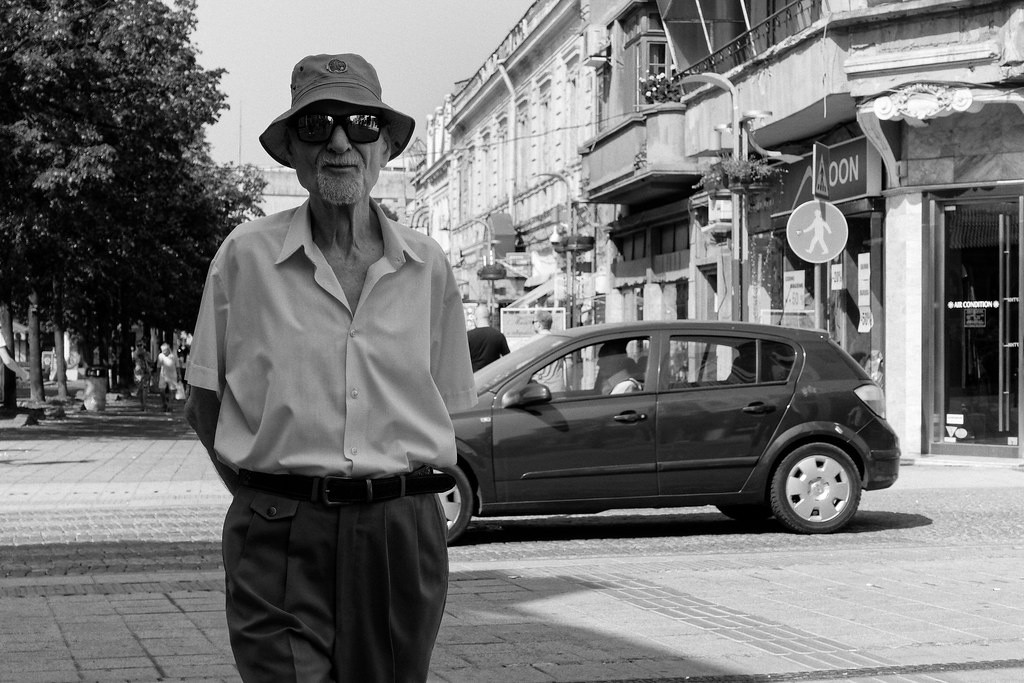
(815, 140), (830, 197)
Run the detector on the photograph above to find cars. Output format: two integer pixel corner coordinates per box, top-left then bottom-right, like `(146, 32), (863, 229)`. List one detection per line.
(428, 321), (902, 550)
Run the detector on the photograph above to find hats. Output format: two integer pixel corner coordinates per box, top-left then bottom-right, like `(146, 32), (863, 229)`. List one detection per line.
(260, 54), (416, 168)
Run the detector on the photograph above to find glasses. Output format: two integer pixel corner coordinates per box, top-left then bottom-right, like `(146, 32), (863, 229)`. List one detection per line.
(286, 111), (389, 146)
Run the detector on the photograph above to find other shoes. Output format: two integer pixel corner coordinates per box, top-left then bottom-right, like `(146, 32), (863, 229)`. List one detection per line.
(162, 405), (173, 412)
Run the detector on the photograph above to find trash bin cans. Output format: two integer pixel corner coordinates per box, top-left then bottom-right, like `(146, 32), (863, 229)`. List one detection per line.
(84, 365), (107, 412)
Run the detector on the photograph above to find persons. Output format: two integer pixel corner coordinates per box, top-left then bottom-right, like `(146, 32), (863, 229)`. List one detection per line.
(0, 322), (30, 382)
(597, 343), (642, 394)
(467, 302), (511, 373)
(156, 343), (181, 411)
(131, 340), (154, 410)
(184, 54), (479, 683)
(531, 310), (567, 393)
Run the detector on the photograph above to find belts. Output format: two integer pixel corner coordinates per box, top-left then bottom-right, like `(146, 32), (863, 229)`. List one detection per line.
(238, 467), (456, 503)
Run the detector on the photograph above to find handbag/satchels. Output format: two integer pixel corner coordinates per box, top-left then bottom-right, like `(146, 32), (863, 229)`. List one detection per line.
(176, 381), (187, 400)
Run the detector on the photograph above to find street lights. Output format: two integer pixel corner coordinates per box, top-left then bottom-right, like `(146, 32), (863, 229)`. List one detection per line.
(534, 169), (578, 334)
(468, 218), (501, 308)
(674, 70), (777, 325)
